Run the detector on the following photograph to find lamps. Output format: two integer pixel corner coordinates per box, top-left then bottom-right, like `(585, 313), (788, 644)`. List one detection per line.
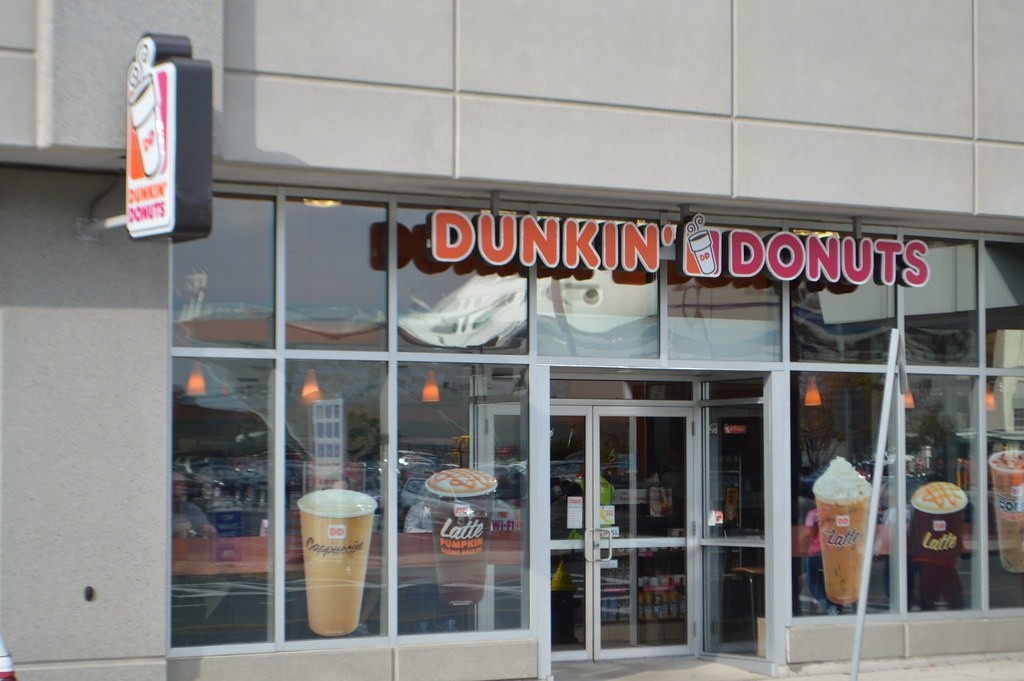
(906, 381), (917, 409)
(302, 367), (323, 400)
(185, 362), (205, 396)
(986, 382), (998, 412)
(421, 369), (442, 402)
(805, 376), (821, 406)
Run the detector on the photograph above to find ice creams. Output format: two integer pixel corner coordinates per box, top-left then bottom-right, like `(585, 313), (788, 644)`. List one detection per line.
(812, 456), (872, 604)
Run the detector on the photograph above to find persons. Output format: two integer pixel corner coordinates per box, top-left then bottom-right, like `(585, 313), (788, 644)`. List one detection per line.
(798, 472), (965, 615)
(173, 463), (217, 537)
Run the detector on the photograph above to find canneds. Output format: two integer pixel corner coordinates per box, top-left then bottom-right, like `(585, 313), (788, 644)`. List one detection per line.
(638, 590), (680, 621)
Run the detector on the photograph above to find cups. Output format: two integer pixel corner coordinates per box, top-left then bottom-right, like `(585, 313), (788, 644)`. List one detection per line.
(912, 497), (968, 604)
(812, 486), (873, 605)
(425, 468), (498, 606)
(988, 450), (1024, 573)
(297, 488), (378, 637)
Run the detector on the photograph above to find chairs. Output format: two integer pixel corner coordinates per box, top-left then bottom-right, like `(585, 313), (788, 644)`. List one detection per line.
(732, 525), (818, 651)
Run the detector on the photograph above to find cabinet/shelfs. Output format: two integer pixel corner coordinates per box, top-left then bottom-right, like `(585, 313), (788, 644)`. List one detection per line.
(709, 446), (760, 582)
